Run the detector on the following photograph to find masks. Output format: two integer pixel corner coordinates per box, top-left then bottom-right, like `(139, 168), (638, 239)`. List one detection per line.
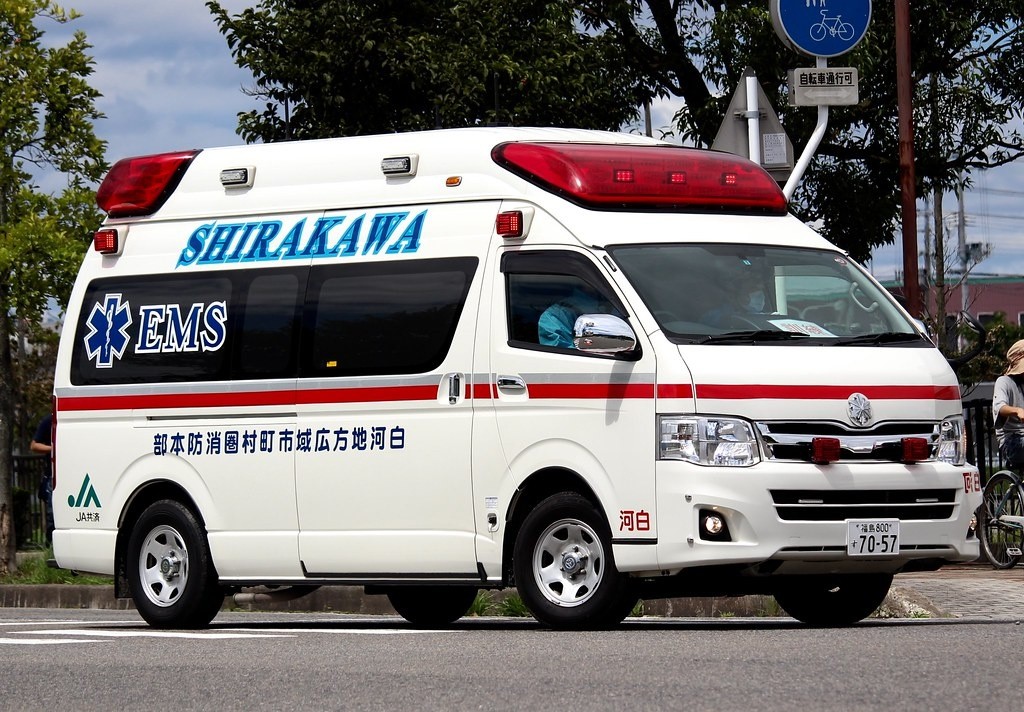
(737, 288), (765, 312)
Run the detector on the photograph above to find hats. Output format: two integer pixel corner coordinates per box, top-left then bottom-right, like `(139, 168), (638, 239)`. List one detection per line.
(725, 257), (776, 286)
(1004, 339), (1024, 375)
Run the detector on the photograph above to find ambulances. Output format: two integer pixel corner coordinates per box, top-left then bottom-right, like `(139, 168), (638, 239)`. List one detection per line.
(51, 126), (984, 630)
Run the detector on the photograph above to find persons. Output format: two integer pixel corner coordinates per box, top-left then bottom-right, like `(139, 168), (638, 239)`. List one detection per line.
(699, 255), (767, 325)
(992, 338), (1024, 472)
(29, 414), (54, 551)
(537, 250), (630, 358)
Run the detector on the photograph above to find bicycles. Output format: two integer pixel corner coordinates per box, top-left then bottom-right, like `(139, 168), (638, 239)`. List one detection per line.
(979, 415), (1024, 570)
